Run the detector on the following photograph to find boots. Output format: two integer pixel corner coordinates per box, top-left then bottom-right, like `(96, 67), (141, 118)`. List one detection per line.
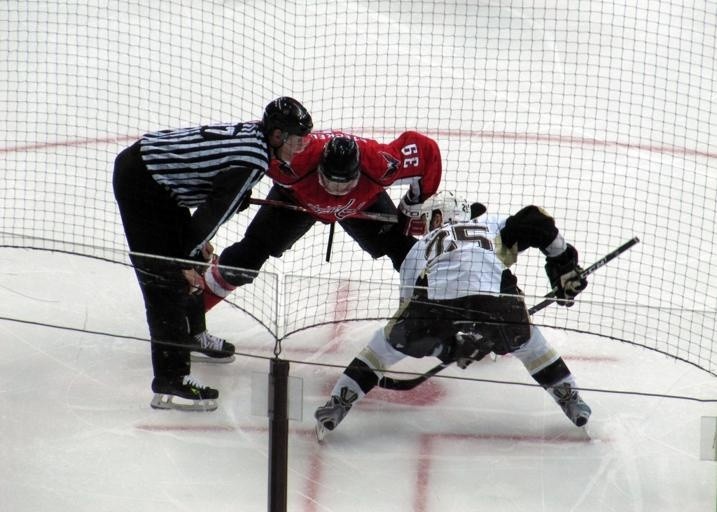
(314, 385), (358, 432)
(549, 384), (592, 427)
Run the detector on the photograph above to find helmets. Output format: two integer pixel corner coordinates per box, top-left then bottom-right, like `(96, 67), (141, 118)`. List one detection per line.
(262, 97), (314, 147)
(317, 135), (361, 184)
(418, 188), (473, 233)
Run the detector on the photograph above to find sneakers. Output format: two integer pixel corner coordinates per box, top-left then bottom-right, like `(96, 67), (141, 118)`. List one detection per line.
(150, 374), (219, 402)
(189, 331), (237, 358)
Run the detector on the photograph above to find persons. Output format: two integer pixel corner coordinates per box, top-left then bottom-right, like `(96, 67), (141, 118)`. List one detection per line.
(315, 190), (592, 431)
(112, 94), (313, 400)
(203, 131), (442, 315)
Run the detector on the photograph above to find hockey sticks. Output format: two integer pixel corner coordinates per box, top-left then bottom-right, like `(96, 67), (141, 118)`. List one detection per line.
(376, 235), (641, 390)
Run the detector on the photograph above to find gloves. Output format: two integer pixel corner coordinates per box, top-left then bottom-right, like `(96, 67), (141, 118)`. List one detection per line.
(396, 180), (425, 231)
(545, 245), (590, 308)
(442, 332), (490, 372)
